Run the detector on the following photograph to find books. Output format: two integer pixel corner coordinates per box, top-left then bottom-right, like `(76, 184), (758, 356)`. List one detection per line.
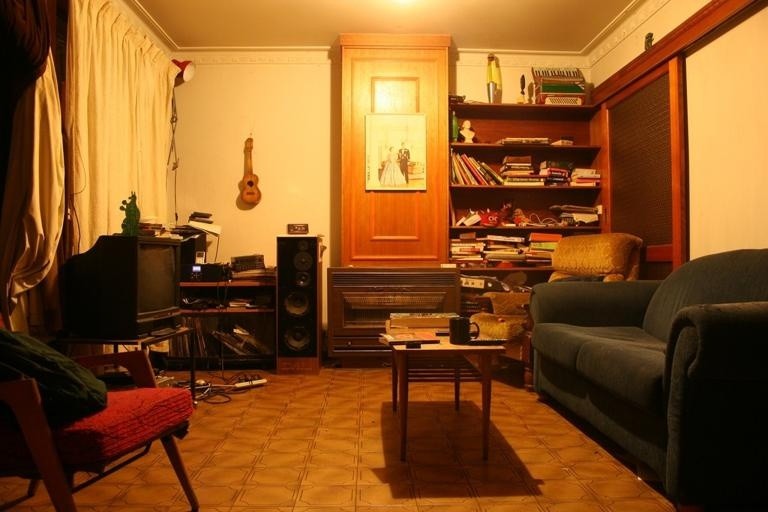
(378, 331), (440, 346)
(140, 211), (274, 356)
(389, 312), (460, 328)
(450, 138), (603, 317)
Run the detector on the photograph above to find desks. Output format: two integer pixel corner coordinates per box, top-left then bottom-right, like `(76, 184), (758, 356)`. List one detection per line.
(64, 328), (196, 393)
(382, 334), (506, 462)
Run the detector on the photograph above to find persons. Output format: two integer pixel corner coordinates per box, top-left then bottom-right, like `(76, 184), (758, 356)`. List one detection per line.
(459, 119), (475, 144)
(379, 145), (407, 185)
(397, 141), (410, 184)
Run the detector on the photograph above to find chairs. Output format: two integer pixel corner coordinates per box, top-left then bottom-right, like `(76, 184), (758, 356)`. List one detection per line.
(0, 353), (201, 512)
(467, 232), (649, 392)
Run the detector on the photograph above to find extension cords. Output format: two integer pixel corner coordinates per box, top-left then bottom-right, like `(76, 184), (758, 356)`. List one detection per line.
(233, 378), (267, 390)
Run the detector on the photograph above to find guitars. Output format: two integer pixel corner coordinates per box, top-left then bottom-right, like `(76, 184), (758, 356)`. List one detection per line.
(241, 138), (261, 204)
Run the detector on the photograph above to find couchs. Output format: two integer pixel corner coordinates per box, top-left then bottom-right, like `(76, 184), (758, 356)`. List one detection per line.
(527, 249), (767, 506)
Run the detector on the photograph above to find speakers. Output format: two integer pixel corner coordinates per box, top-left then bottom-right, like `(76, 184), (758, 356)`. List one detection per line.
(276, 235), (323, 375)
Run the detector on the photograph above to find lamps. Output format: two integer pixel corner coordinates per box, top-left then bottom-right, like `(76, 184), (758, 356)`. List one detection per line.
(170, 58), (196, 82)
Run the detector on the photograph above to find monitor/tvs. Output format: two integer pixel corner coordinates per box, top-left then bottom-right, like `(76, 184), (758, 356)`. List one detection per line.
(72, 235), (181, 341)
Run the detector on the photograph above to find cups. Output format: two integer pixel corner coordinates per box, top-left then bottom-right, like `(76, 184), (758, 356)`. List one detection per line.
(449, 318), (480, 346)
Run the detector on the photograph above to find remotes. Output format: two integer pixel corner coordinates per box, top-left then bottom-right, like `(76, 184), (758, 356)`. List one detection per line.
(469, 338), (508, 345)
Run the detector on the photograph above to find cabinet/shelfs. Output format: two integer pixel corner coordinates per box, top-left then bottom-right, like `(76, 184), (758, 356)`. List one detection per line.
(171, 275), (274, 370)
(448, 97), (602, 319)
